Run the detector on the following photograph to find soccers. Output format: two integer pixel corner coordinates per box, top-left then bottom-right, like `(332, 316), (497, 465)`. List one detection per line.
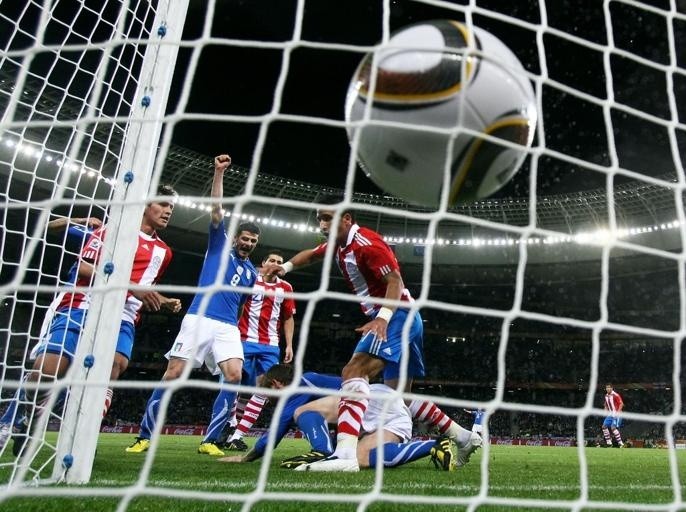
(344, 17), (538, 208)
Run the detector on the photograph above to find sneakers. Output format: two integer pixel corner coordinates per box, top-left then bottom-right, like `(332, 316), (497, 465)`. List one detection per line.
(279, 453), (326, 470)
(432, 439), (451, 468)
(456, 433), (484, 463)
(124, 440), (153, 454)
(222, 439), (248, 451)
(198, 442), (225, 456)
(292, 457), (363, 474)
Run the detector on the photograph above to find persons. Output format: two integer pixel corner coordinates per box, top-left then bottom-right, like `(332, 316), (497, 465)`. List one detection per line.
(490, 386), (601, 447)
(164, 367), (214, 425)
(602, 383), (628, 447)
(464, 407), (489, 436)
(454, 405), (474, 430)
(0, 216), (101, 452)
(217, 365), (453, 471)
(11, 186), (181, 459)
(288, 263), (365, 377)
(221, 249), (294, 451)
(402, 255), (686, 384)
(107, 301), (177, 424)
(628, 385), (685, 448)
(264, 193), (424, 472)
(125, 153), (258, 458)
(425, 385), (496, 402)
